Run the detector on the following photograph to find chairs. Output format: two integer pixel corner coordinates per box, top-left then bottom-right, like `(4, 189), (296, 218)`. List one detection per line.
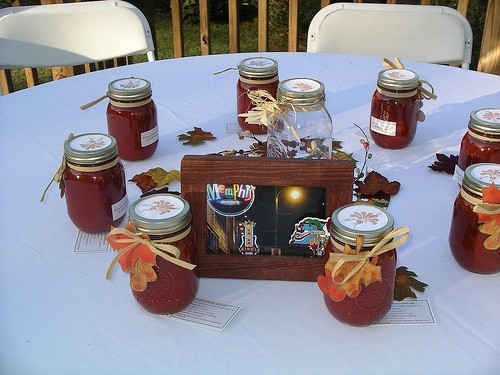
(0, 0), (156, 97)
(306, 2), (473, 70)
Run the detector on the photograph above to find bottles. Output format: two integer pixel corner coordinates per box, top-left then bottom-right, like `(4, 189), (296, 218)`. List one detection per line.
(129, 193), (200, 315)
(448, 162), (500, 275)
(237, 57), (280, 135)
(106, 77), (160, 161)
(318, 203), (397, 328)
(370, 68), (421, 150)
(62, 132), (127, 234)
(266, 78), (333, 160)
(456, 107), (500, 187)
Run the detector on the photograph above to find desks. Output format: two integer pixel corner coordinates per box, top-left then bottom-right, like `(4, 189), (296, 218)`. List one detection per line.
(0, 52), (500, 375)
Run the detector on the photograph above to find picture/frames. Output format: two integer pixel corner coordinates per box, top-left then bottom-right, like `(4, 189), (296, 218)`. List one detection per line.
(181, 154), (354, 283)
